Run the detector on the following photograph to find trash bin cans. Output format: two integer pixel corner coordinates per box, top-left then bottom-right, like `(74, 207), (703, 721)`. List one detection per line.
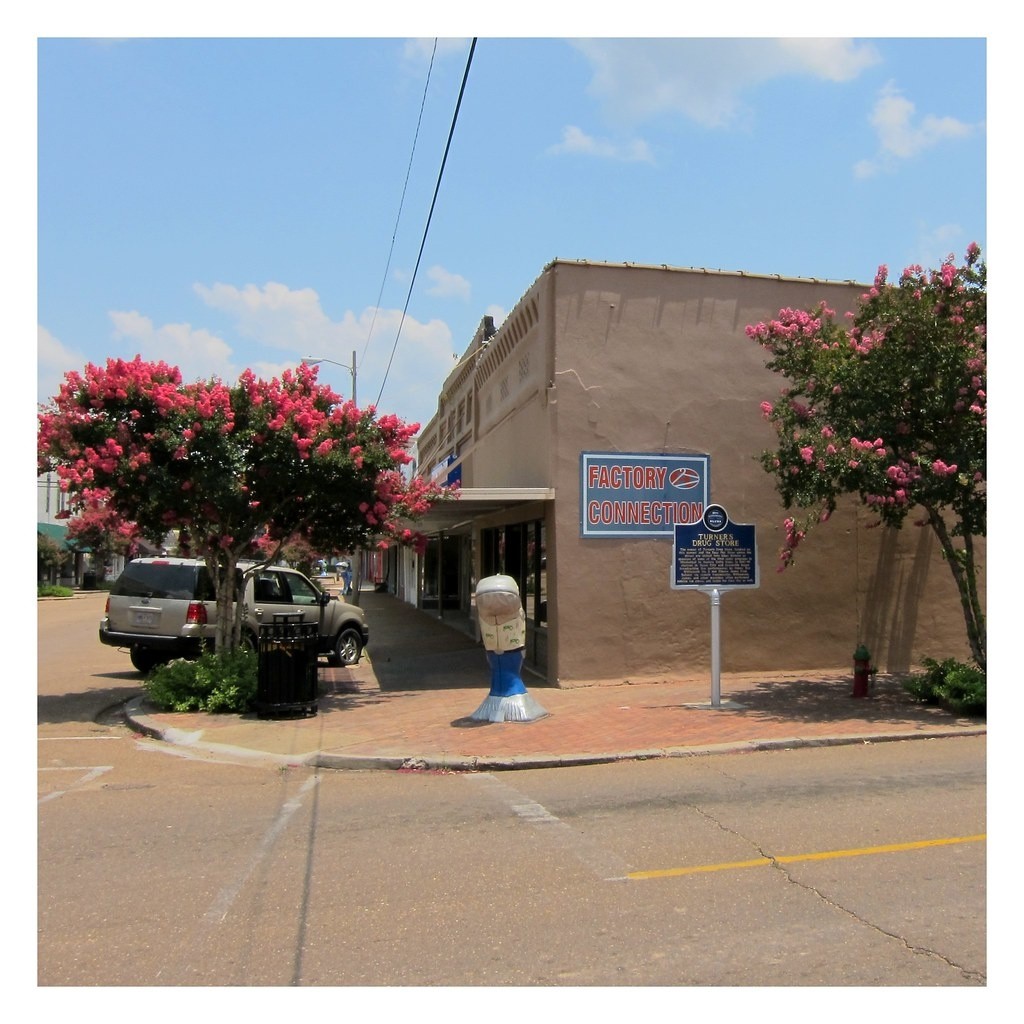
(254, 610), (321, 720)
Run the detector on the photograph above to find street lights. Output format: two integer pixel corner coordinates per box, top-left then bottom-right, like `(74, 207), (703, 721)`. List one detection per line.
(299, 348), (357, 404)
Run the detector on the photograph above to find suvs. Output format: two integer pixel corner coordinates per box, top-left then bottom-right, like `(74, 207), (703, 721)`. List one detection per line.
(100, 555), (369, 676)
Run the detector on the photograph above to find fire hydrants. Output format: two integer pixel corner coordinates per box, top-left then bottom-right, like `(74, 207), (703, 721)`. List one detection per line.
(849, 644), (879, 699)
